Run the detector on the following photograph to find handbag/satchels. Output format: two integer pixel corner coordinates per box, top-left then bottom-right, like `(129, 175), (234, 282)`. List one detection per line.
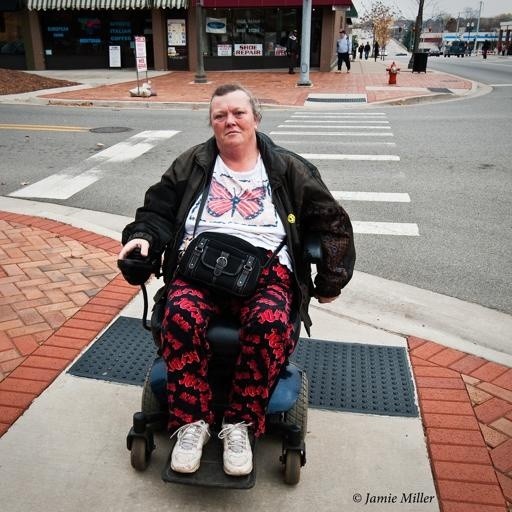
(180, 148), (288, 303)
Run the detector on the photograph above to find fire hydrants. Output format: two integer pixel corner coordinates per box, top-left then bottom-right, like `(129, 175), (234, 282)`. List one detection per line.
(386, 60), (401, 84)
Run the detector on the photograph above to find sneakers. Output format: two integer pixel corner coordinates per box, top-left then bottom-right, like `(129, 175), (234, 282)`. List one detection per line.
(218, 416), (256, 478)
(334, 69), (351, 75)
(167, 418), (211, 476)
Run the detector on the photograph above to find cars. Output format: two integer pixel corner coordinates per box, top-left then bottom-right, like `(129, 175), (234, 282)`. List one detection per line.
(444, 39), (466, 57)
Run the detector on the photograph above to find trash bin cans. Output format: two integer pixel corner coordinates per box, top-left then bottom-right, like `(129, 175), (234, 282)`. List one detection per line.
(413, 52), (428, 73)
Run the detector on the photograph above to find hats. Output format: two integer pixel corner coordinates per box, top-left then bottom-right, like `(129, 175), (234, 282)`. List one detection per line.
(339, 30), (346, 34)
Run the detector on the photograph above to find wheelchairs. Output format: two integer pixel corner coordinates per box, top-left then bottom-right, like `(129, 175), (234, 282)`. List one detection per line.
(117, 240), (322, 489)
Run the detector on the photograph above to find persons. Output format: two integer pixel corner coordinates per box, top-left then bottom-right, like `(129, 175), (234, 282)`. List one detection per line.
(352, 44), (356, 59)
(502, 44), (505, 56)
(364, 42), (370, 60)
(482, 40), (489, 59)
(335, 31), (352, 73)
(359, 43), (364, 59)
(373, 42), (380, 62)
(118, 84), (356, 476)
(287, 30), (298, 74)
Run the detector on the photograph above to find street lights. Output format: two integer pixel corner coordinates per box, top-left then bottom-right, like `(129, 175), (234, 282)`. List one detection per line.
(466, 22), (474, 40)
